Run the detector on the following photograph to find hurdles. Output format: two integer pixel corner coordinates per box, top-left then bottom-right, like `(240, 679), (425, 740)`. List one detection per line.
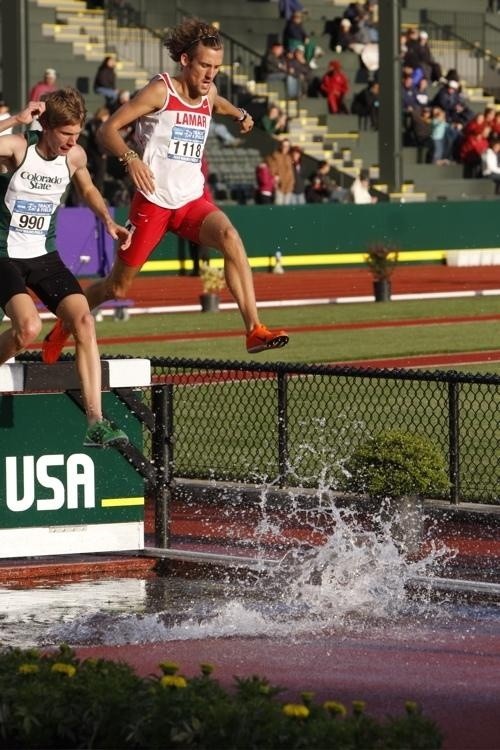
(1, 359), (174, 562)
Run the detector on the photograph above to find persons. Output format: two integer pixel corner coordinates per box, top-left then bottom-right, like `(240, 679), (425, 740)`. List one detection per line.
(1, 57), (133, 197)
(41, 18), (288, 366)
(255, 139), (378, 206)
(0, 88), (133, 449)
(260, 0), (500, 181)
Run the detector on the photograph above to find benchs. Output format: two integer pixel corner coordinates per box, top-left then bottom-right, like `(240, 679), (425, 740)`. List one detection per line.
(29, 0), (500, 204)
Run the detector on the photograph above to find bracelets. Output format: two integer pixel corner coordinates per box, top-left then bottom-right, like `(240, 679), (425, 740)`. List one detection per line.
(119, 150), (139, 167)
(238, 108), (247, 122)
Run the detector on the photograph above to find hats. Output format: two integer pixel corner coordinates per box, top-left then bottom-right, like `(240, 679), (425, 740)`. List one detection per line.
(261, 13), (458, 159)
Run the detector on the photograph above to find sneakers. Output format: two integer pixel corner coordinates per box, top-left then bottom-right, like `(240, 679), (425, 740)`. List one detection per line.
(41, 318), (73, 363)
(84, 421), (128, 448)
(246, 326), (289, 353)
